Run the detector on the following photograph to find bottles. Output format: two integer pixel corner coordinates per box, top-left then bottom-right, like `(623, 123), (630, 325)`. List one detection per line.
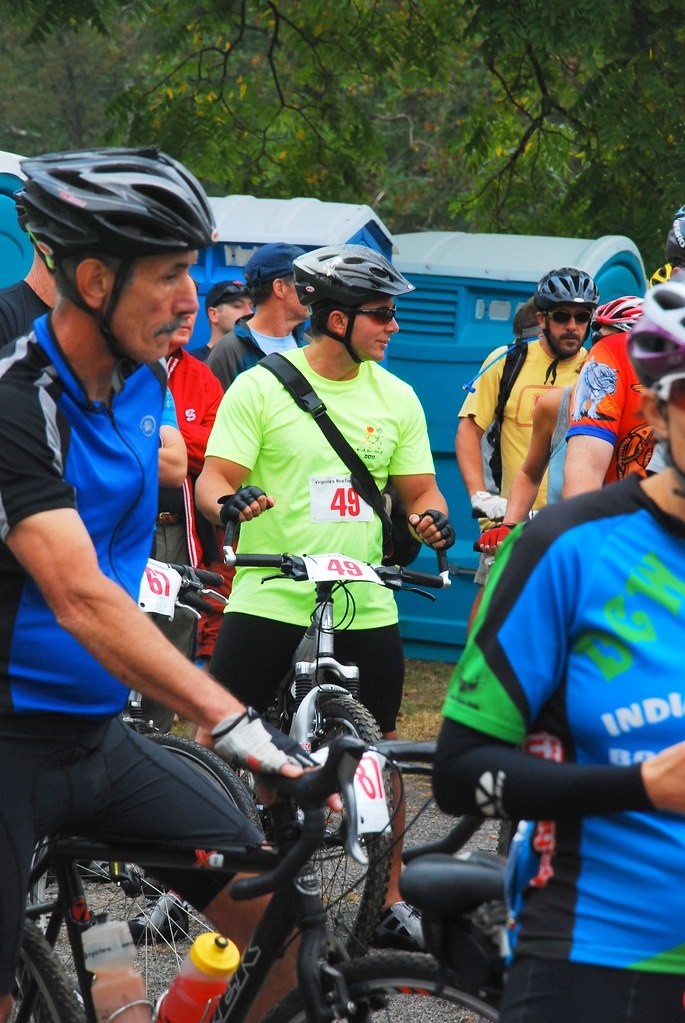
(81, 910), (152, 1022)
(156, 932), (243, 1023)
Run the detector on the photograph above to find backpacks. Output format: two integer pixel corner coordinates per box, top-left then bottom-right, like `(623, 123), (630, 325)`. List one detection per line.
(480, 337), (527, 493)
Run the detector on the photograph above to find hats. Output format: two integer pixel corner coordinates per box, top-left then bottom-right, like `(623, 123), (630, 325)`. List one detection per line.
(244, 242), (306, 282)
(206, 280), (251, 312)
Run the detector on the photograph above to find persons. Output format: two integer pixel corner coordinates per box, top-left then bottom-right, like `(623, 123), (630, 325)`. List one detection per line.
(664, 205), (685, 266)
(430, 267), (685, 1023)
(0, 143), (305, 1023)
(195, 241), (456, 953)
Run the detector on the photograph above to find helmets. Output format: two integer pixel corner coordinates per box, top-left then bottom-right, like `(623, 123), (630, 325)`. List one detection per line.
(534, 267), (600, 316)
(649, 263), (672, 288)
(590, 296), (644, 331)
(629, 281), (685, 390)
(19, 145), (219, 275)
(665, 205), (685, 266)
(292, 244), (416, 306)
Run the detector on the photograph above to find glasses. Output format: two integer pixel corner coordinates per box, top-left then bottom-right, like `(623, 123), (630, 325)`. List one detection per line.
(591, 331), (603, 343)
(329, 304), (396, 323)
(543, 310), (593, 324)
(650, 371), (685, 412)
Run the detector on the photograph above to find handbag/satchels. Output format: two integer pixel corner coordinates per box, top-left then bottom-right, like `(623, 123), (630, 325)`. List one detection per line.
(381, 479), (422, 567)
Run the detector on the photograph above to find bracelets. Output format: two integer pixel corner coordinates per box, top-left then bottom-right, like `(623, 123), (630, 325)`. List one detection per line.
(107, 999), (155, 1023)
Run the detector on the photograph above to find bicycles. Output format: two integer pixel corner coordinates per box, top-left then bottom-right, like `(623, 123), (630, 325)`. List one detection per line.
(12, 508), (517, 1023)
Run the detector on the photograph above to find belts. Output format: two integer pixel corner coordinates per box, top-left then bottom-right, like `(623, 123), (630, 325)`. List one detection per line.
(156, 512), (185, 525)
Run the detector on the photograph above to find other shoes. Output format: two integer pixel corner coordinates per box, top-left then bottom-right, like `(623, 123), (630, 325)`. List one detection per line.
(374, 901), (425, 951)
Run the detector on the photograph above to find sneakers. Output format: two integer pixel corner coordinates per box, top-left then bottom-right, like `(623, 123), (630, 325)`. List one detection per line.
(127, 894), (188, 943)
